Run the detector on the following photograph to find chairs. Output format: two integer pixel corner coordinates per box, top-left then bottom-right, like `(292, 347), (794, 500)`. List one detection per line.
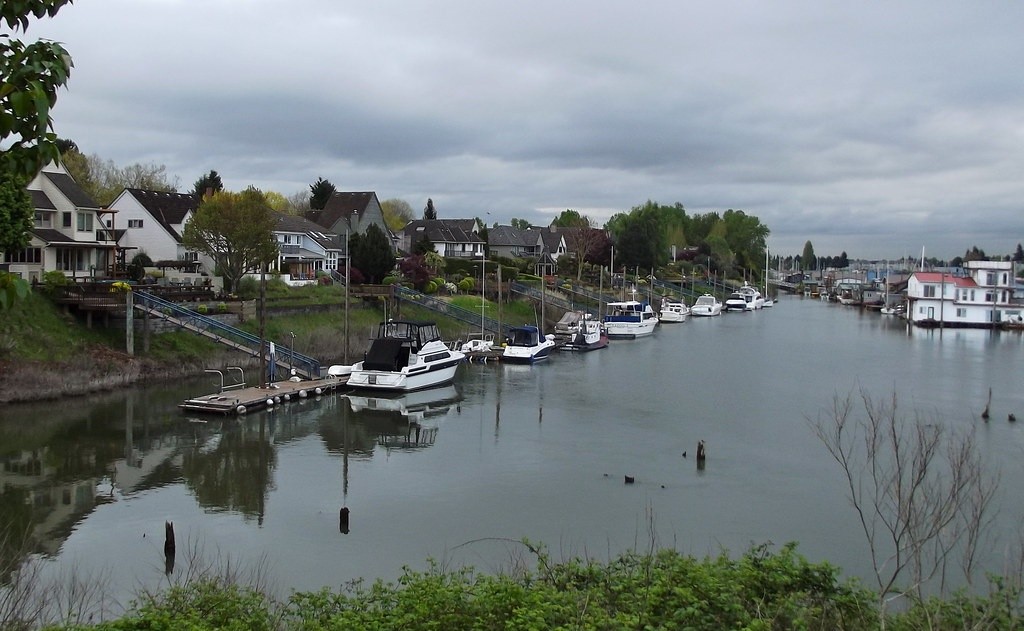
(172, 276), (203, 289)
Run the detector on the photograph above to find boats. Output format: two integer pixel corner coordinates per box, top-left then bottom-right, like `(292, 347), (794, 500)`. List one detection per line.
(879, 259), (905, 315)
(726, 281), (764, 311)
(345, 318), (467, 393)
(604, 300), (659, 337)
(460, 337), (505, 358)
(339, 381), (466, 452)
(559, 311), (609, 352)
(837, 289), (862, 305)
(503, 325), (556, 361)
(659, 301), (688, 323)
(691, 293), (722, 316)
